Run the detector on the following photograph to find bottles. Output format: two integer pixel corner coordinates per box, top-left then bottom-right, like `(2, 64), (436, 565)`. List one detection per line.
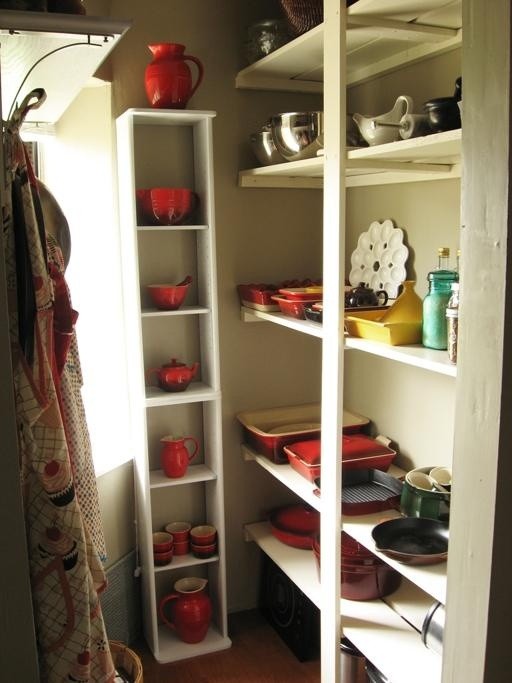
(422, 270), (457, 350)
(437, 247), (450, 270)
(446, 280), (458, 364)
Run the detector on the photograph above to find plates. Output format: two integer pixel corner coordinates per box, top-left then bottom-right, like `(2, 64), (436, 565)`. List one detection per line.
(282, 435), (398, 482)
(236, 282), (281, 312)
(235, 400), (371, 464)
(271, 294), (323, 319)
(344, 309), (423, 345)
(270, 506), (319, 551)
(279, 286), (351, 298)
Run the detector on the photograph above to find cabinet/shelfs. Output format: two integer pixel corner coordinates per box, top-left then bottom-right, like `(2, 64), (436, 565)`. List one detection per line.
(234, 2), (511, 683)
(117, 107), (233, 664)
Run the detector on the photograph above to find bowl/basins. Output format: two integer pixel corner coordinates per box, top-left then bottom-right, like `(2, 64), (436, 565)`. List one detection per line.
(270, 111), (357, 161)
(422, 96), (462, 132)
(154, 548), (173, 566)
(401, 467), (451, 522)
(147, 276), (193, 310)
(166, 522), (191, 541)
(190, 525), (216, 542)
(152, 532), (173, 552)
(191, 544), (217, 558)
(248, 131), (287, 166)
(173, 543), (190, 555)
(137, 188), (200, 224)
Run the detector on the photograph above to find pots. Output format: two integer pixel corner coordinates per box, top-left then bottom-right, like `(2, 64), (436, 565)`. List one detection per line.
(372, 516), (449, 565)
(310, 466), (412, 516)
(313, 532), (402, 603)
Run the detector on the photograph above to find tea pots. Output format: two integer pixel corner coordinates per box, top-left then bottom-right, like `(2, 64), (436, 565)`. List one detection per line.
(160, 576), (211, 644)
(345, 281), (388, 305)
(160, 434), (199, 479)
(146, 359), (200, 393)
(145, 43), (205, 108)
